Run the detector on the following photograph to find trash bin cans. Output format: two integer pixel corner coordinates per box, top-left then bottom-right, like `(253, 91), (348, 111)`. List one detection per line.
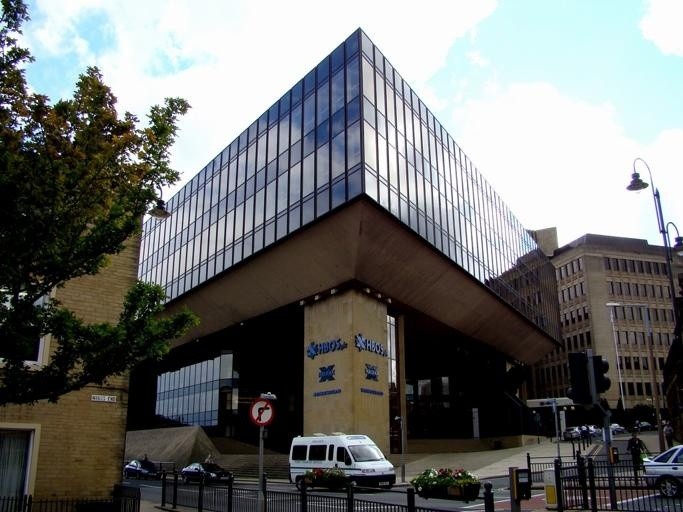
(113, 483), (141, 512)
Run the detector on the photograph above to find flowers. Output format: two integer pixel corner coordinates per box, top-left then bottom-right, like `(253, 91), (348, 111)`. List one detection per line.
(409, 467), (482, 486)
(304, 466), (348, 480)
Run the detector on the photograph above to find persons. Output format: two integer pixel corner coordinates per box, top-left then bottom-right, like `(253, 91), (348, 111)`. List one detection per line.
(143, 453), (148, 460)
(204, 454), (214, 463)
(662, 421), (674, 449)
(625, 429), (643, 467)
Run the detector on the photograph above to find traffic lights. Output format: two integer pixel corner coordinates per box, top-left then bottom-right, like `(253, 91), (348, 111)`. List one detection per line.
(568, 351), (611, 403)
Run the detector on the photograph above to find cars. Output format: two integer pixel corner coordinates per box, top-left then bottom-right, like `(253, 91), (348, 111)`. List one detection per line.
(124, 459), (162, 481)
(564, 422), (625, 441)
(642, 445), (683, 497)
(180, 462), (233, 486)
(634, 421), (651, 432)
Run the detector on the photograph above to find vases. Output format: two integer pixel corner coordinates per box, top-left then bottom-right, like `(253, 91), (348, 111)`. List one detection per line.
(309, 480), (348, 489)
(415, 486), (482, 501)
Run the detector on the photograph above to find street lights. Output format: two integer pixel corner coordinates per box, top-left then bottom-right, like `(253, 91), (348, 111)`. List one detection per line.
(606, 302), (665, 451)
(626, 157), (682, 340)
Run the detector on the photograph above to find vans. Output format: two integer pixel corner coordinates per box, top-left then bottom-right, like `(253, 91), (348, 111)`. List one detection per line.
(289, 432), (396, 491)
